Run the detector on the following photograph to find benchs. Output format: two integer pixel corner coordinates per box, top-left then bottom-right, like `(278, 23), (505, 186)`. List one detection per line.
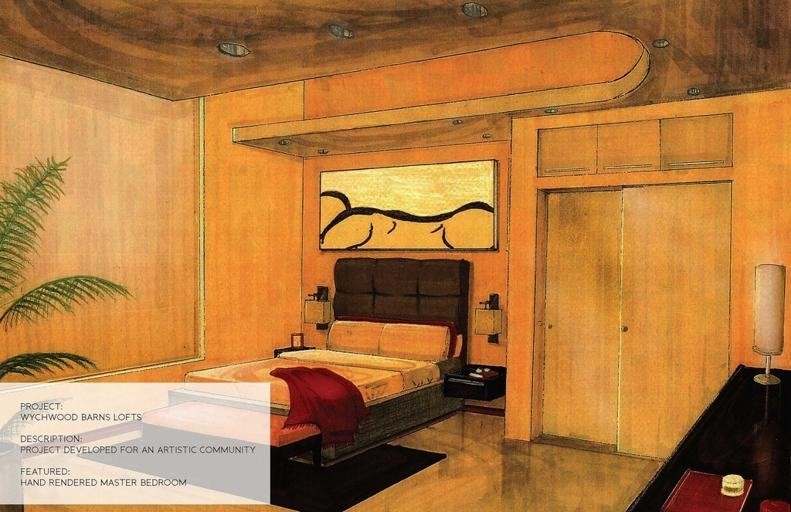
(142, 401), (323, 484)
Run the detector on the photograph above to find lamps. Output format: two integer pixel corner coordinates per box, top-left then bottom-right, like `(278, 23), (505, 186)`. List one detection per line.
(304, 286), (331, 330)
(475, 294), (503, 344)
(752, 264), (786, 386)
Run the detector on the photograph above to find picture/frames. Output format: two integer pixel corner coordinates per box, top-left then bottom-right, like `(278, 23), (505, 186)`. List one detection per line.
(291, 333), (304, 350)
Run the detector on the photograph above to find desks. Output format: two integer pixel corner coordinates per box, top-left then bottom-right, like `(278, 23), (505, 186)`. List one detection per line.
(625, 364), (790, 512)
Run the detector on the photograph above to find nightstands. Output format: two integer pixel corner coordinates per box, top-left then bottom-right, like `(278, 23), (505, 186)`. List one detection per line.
(443, 364), (506, 400)
(274, 346), (315, 358)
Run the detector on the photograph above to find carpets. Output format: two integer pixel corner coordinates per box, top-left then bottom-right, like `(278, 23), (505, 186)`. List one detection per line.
(77, 436), (447, 512)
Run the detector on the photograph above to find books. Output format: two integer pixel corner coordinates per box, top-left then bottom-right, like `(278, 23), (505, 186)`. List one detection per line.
(660, 468), (755, 512)
(469, 370), (500, 378)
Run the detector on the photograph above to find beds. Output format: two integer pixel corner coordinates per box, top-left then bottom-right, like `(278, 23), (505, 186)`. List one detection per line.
(168, 258), (471, 462)
